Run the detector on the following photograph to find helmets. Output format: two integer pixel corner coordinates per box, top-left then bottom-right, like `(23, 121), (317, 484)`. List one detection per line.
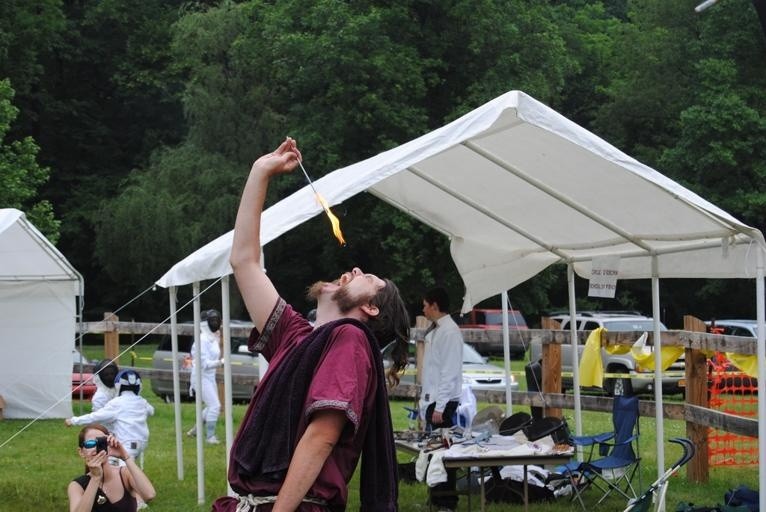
(200, 309), (221, 334)
(115, 368), (142, 396)
(93, 359), (118, 389)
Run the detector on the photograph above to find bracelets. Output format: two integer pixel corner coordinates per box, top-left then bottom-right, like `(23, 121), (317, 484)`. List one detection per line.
(123, 456), (130, 462)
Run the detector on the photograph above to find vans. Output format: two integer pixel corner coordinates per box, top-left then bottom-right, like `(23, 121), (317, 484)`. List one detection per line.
(151, 320), (260, 403)
(425, 310), (528, 361)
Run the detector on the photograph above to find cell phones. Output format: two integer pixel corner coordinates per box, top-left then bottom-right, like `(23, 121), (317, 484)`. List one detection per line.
(96, 436), (108, 456)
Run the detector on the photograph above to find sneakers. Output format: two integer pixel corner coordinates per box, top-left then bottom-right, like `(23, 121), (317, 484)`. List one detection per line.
(186, 429), (197, 437)
(205, 436), (219, 443)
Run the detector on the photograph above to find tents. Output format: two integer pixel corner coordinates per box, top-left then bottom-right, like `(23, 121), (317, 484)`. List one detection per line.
(1, 207), (86, 420)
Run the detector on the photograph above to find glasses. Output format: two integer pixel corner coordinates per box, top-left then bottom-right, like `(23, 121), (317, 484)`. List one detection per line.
(82, 440), (96, 449)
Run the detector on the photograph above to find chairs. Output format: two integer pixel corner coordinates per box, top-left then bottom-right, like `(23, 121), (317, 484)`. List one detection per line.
(552, 395), (643, 510)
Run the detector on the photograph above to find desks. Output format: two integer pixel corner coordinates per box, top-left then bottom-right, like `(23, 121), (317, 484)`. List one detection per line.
(395, 433), (574, 511)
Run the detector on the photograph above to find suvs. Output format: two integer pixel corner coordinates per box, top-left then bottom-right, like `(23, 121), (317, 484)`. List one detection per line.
(531, 309), (685, 398)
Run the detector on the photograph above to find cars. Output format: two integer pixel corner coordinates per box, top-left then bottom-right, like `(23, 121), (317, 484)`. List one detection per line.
(380, 341), (520, 391)
(72, 362), (99, 397)
(703, 319), (757, 337)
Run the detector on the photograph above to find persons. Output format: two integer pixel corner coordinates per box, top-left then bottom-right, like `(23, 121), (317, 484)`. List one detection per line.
(187, 310), (225, 445)
(67, 424), (157, 512)
(91, 359), (119, 413)
(213, 136), (412, 512)
(64, 368), (155, 512)
(418, 287), (464, 512)
